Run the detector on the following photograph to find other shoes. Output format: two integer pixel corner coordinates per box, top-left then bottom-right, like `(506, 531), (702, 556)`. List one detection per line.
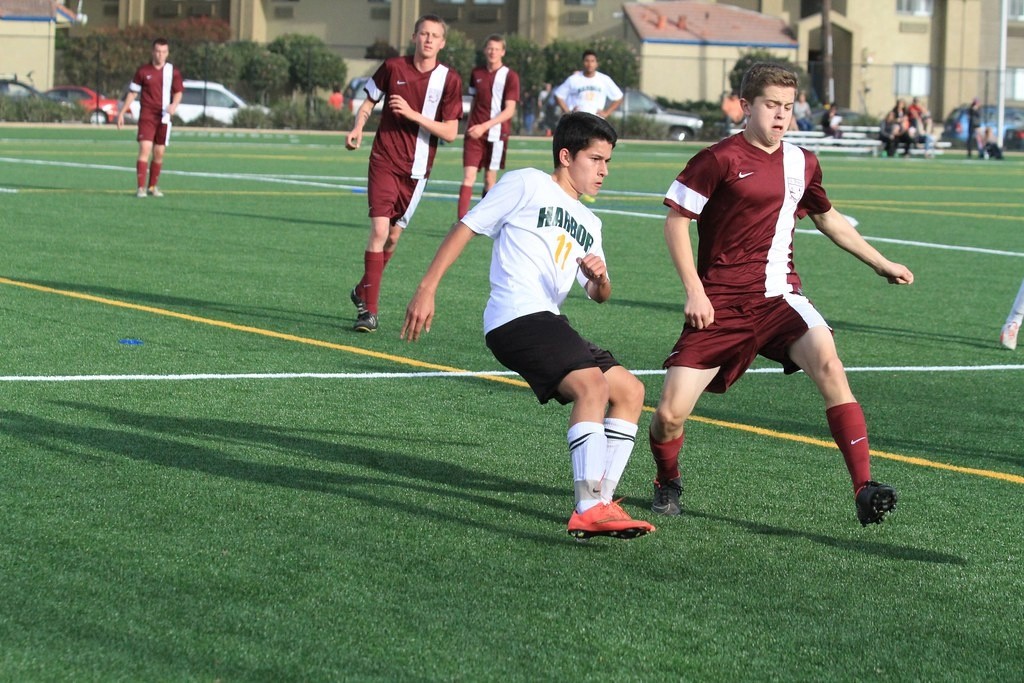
(525, 130), (533, 136)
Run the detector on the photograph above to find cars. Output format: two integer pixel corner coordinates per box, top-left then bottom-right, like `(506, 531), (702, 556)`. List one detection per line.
(0, 79), (73, 108)
(43, 86), (119, 125)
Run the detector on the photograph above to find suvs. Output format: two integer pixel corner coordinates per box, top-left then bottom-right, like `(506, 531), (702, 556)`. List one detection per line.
(341, 78), (473, 118)
(941, 106), (1024, 148)
(546, 84), (704, 142)
(119, 80), (270, 130)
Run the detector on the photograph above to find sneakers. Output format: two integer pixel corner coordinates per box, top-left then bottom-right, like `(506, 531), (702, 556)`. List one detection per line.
(581, 195), (595, 202)
(855, 480), (898, 528)
(148, 186), (163, 197)
(568, 494), (655, 543)
(1000, 322), (1019, 349)
(350, 284), (379, 332)
(137, 186), (147, 197)
(652, 470), (684, 515)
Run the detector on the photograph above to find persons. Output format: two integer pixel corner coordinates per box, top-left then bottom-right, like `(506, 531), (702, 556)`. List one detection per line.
(517, 69), (579, 136)
(457, 35), (522, 218)
(117, 38), (184, 197)
(964, 98), (1004, 160)
(554, 51), (625, 121)
(327, 86), (344, 111)
(649, 63), (913, 525)
(344, 14), (464, 332)
(400, 111), (655, 540)
(720, 89), (843, 137)
(1000, 278), (1024, 351)
(879, 95), (938, 160)
